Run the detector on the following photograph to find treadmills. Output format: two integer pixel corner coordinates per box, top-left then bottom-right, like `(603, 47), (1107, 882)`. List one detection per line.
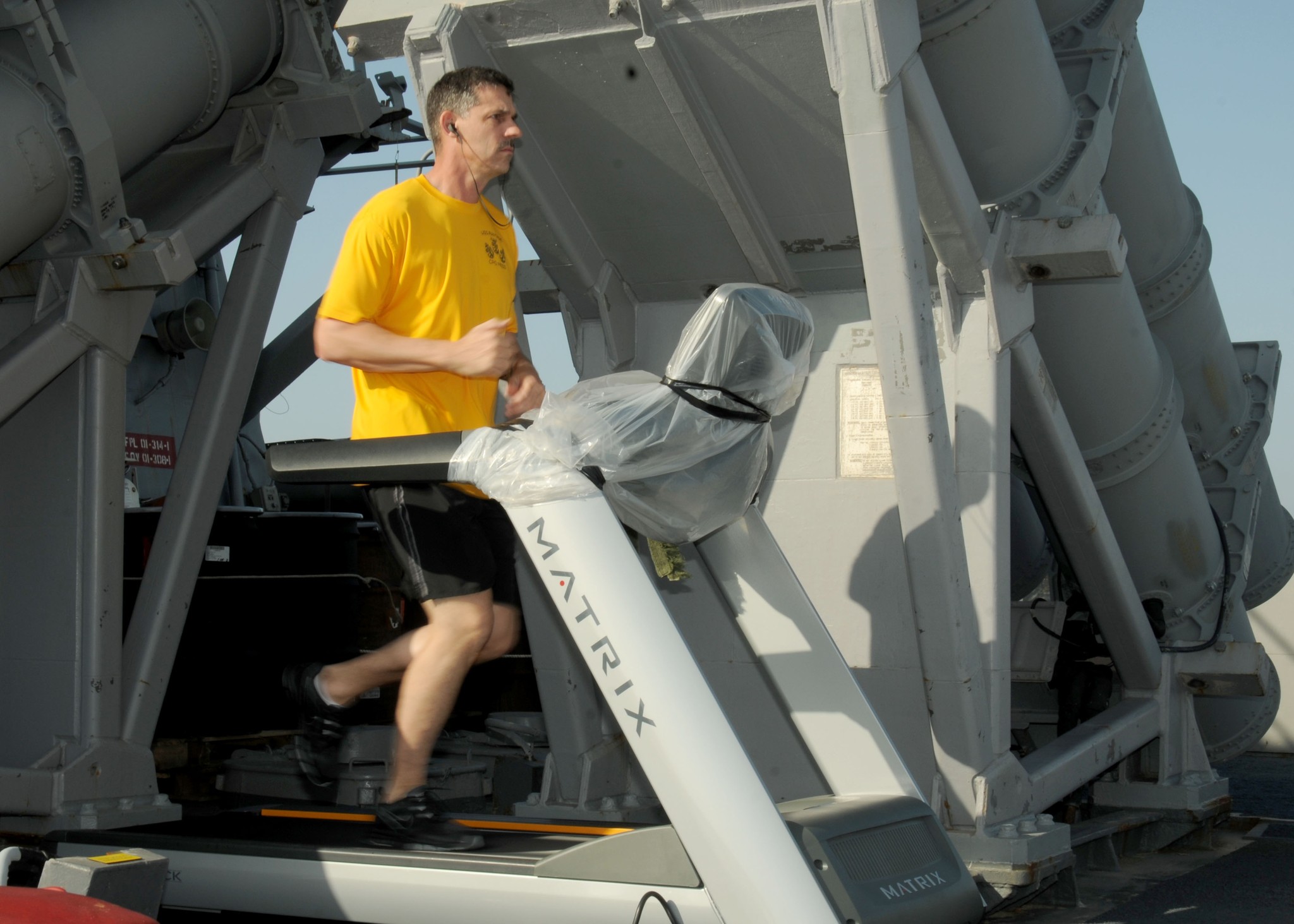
(40, 280), (986, 924)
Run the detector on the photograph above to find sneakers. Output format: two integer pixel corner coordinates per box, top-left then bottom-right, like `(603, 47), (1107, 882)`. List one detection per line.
(282, 661), (354, 789)
(366, 786), (485, 852)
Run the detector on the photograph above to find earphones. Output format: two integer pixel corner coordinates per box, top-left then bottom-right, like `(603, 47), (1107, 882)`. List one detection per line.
(447, 123), (459, 137)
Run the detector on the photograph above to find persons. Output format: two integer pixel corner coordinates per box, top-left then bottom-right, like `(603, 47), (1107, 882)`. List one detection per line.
(295, 68), (547, 857)
